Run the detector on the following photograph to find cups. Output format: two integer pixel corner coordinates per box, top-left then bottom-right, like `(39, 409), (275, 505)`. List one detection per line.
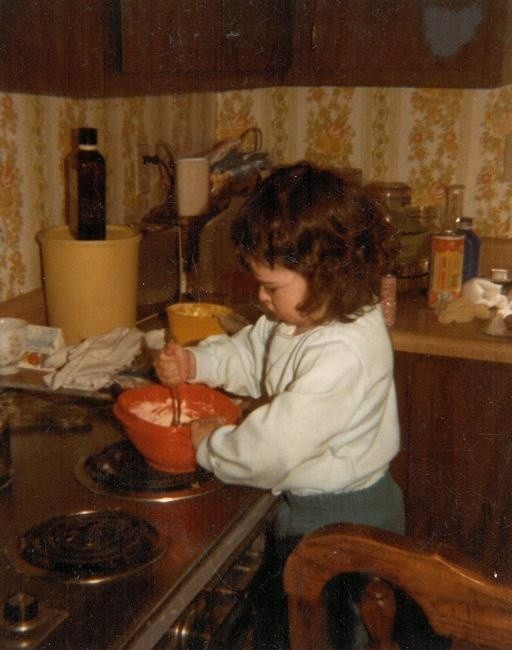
(177, 157), (209, 217)
(0, 318), (29, 375)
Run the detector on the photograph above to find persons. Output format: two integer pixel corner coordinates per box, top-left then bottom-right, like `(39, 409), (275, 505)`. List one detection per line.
(153, 160), (408, 649)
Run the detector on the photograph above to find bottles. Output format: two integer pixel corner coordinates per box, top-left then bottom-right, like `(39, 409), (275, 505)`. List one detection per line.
(67, 128), (108, 238)
(430, 219), (479, 305)
(381, 267), (396, 325)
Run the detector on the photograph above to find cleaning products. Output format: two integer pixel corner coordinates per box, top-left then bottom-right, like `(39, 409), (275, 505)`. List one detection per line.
(61, 124), (109, 239)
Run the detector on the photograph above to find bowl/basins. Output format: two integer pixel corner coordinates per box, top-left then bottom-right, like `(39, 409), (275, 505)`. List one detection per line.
(165, 302), (234, 343)
(114, 383), (241, 475)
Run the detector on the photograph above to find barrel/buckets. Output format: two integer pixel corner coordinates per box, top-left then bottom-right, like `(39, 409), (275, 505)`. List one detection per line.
(201, 186), (253, 299)
(37, 224), (143, 344)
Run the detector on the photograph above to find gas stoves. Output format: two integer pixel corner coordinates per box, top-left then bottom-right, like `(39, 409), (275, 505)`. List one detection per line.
(0, 385), (283, 645)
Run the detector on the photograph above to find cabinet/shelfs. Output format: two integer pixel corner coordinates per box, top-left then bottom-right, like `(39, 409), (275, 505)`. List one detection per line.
(111, 1), (290, 81)
(390, 351), (511, 589)
(296, 2), (482, 74)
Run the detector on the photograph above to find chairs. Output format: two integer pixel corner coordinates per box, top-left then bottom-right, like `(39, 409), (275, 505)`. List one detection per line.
(280, 519), (512, 650)
